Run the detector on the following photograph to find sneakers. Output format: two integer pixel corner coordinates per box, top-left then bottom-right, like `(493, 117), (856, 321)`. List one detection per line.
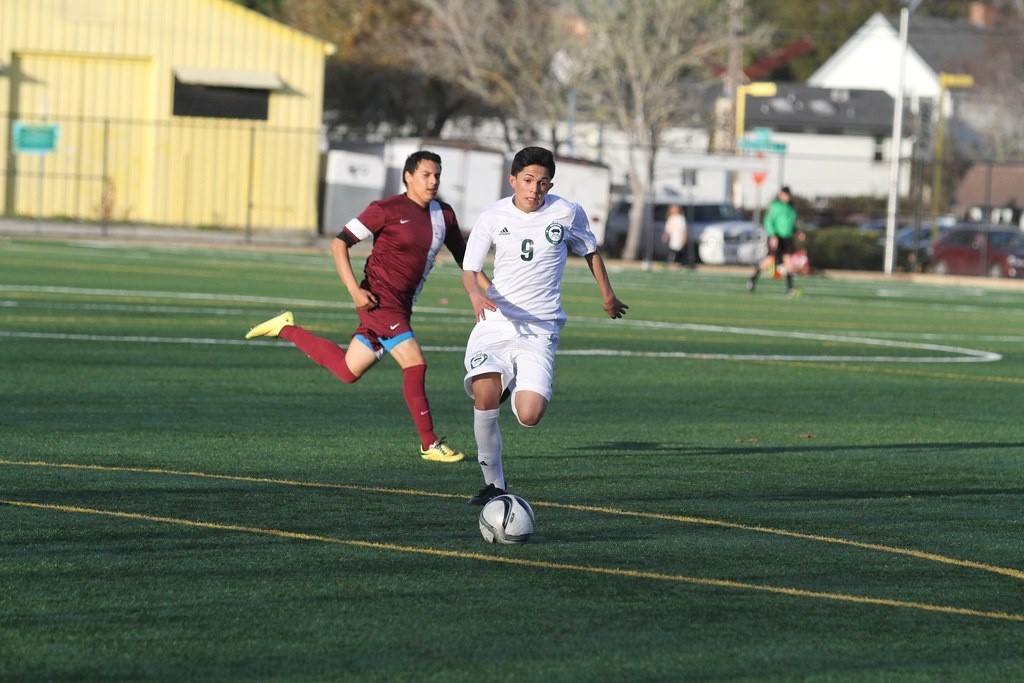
(420, 436), (464, 462)
(244, 311), (295, 339)
(499, 387), (511, 404)
(468, 481), (509, 506)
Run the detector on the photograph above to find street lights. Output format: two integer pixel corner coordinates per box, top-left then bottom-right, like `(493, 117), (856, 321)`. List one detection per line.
(734, 79), (776, 157)
(932, 72), (974, 231)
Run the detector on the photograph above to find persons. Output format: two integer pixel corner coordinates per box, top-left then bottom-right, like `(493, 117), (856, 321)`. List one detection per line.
(662, 203), (687, 266)
(746, 186), (806, 296)
(461, 147), (629, 505)
(244, 151), (490, 462)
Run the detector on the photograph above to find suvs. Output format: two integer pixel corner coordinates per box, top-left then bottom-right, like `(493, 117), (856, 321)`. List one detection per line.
(605, 197), (773, 268)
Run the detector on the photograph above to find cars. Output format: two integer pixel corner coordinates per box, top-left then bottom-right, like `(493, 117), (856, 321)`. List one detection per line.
(895, 227), (953, 271)
(928, 228), (1024, 278)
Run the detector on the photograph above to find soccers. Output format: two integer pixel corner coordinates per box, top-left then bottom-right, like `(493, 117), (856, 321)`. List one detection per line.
(478, 494), (536, 545)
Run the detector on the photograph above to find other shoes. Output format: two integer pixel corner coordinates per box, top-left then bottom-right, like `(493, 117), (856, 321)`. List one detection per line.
(786, 288), (798, 295)
(746, 276), (756, 293)
